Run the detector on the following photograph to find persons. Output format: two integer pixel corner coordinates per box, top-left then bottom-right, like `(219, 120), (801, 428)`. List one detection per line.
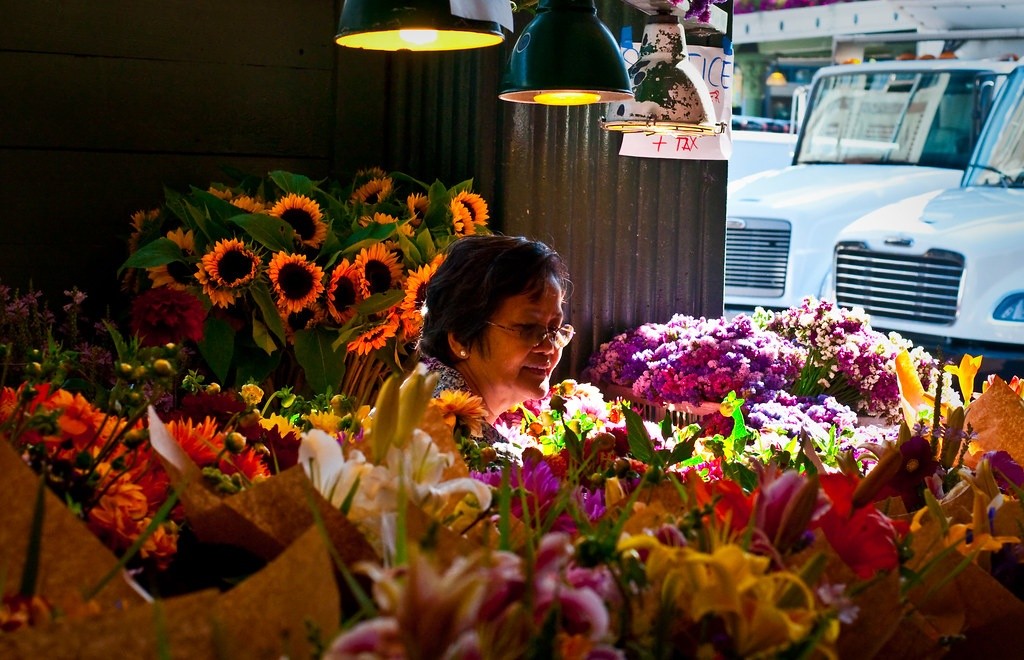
(366, 235), (575, 472)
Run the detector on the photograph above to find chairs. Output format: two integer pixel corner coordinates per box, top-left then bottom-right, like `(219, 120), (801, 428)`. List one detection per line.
(924, 126), (959, 156)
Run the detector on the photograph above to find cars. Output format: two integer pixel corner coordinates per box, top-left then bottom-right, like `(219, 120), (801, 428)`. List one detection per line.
(725, 62), (1024, 391)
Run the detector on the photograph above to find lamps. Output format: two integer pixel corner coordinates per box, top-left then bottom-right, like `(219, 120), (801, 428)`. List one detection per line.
(332, 0), (506, 51)
(765, 58), (787, 86)
(497, 0), (633, 106)
(600, 0), (717, 137)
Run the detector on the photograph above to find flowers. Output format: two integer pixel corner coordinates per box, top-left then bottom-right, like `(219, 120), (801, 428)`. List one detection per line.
(0, 167), (1024, 660)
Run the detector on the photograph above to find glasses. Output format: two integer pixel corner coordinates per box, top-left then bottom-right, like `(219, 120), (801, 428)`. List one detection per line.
(484, 320), (576, 347)
